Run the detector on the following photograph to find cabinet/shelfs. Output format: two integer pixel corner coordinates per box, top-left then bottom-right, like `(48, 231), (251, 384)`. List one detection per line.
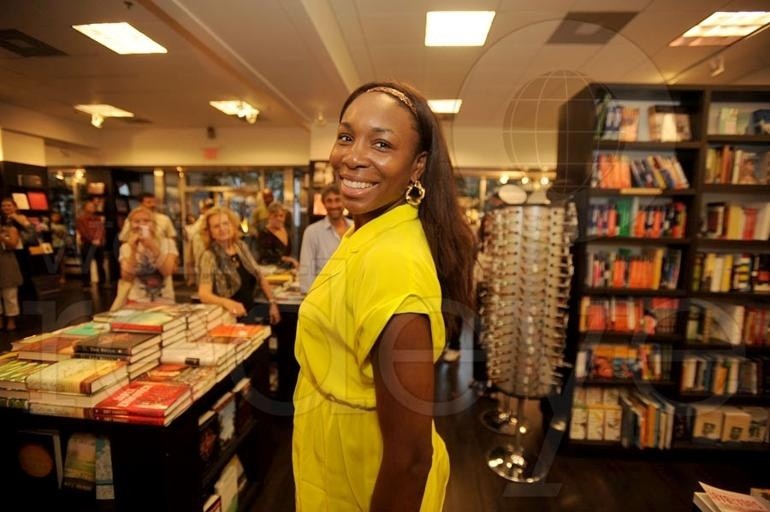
(685, 85), (770, 463)
(557, 83), (706, 463)
(1, 325), (276, 512)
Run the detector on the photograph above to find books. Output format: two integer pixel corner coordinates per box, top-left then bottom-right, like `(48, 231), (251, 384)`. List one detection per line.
(569, 104), (770, 512)
(1, 302), (275, 512)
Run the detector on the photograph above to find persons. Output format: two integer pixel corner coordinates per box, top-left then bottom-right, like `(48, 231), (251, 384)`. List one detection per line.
(284, 82), (477, 512)
(1, 182), (359, 380)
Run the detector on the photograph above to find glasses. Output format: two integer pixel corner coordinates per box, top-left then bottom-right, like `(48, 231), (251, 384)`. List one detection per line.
(478, 203), (578, 400)
